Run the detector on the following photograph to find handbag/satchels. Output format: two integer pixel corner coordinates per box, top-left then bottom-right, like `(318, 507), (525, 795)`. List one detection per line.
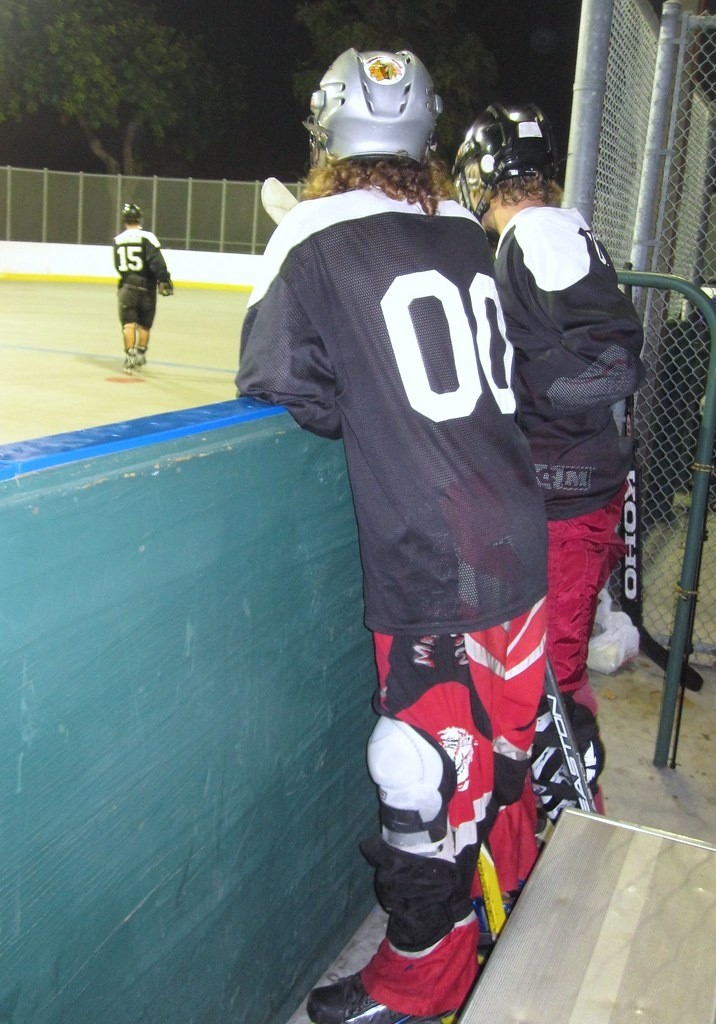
(587, 587), (640, 675)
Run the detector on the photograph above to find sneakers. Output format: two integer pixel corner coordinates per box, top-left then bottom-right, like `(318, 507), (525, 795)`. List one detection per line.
(123, 347), (134, 376)
(471, 894), (524, 945)
(308, 970), (456, 1024)
(137, 346), (148, 370)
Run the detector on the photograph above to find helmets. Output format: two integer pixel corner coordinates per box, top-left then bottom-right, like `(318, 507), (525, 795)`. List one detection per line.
(310, 47), (442, 162)
(451, 101), (553, 189)
(120, 203), (142, 221)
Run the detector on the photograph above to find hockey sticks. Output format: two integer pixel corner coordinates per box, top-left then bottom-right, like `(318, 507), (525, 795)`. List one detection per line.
(467, 828), (506, 944)
(620, 262), (705, 693)
(255, 179), (607, 822)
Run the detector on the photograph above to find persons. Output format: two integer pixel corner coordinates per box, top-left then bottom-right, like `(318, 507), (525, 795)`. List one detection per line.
(234, 47), (550, 1024)
(113, 203), (174, 374)
(451, 100), (645, 826)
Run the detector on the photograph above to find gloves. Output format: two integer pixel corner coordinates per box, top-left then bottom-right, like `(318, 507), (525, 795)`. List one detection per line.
(159, 280), (173, 297)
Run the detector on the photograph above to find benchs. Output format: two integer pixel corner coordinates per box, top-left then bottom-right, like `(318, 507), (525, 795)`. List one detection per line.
(455, 807), (716, 1024)
